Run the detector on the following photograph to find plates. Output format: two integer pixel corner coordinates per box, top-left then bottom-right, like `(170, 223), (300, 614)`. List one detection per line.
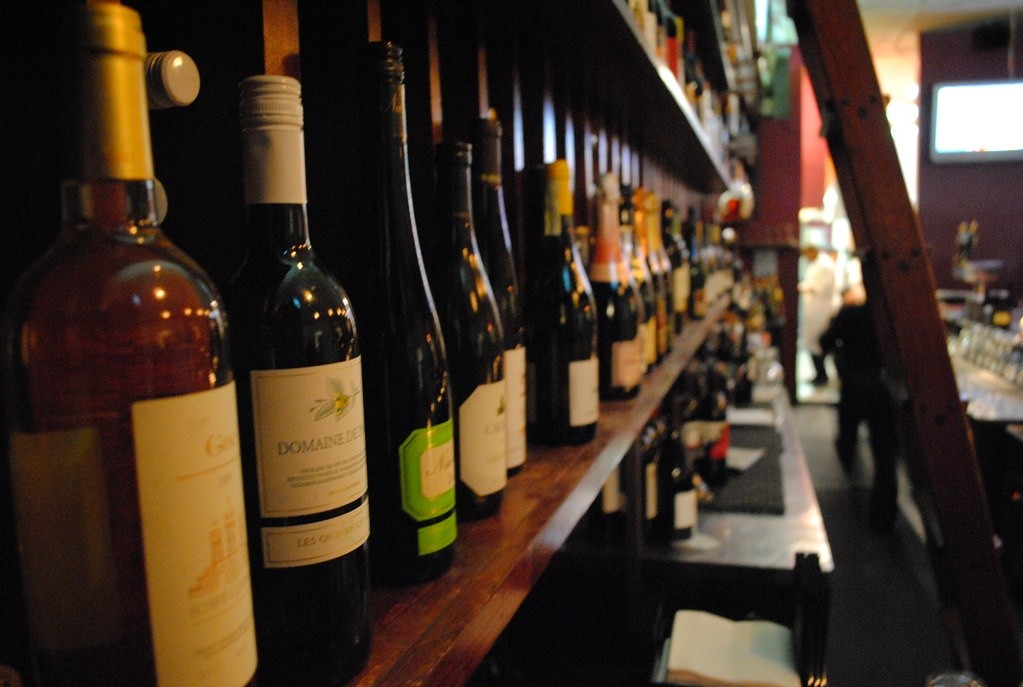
(226, 70), (373, 686)
(0, 0), (274, 686)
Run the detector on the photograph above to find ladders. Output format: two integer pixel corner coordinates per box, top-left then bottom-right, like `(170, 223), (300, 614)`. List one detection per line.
(784, 0), (1023, 687)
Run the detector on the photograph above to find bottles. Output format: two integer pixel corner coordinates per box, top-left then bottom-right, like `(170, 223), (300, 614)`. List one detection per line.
(354, 0), (779, 597)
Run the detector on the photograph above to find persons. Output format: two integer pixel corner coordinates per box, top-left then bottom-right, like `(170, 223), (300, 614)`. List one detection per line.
(797, 244), (896, 531)
(970, 259), (1013, 331)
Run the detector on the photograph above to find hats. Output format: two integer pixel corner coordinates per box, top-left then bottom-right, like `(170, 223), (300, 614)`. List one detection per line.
(800, 229), (821, 249)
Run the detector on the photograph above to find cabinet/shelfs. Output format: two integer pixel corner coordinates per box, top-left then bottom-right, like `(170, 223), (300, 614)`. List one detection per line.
(0, 0), (745, 687)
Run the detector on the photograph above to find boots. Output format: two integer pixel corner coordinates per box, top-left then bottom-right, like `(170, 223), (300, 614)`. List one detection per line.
(812, 355), (827, 385)
